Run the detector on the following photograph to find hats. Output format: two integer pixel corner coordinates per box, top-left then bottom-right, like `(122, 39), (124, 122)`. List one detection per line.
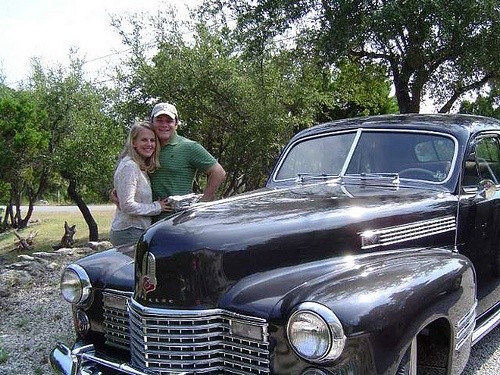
(151, 103), (178, 119)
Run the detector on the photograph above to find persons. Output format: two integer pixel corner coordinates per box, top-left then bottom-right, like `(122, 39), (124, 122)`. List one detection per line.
(109, 123), (175, 246)
(111, 102), (225, 224)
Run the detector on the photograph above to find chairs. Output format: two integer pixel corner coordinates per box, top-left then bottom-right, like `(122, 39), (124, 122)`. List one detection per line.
(399, 160), (500, 187)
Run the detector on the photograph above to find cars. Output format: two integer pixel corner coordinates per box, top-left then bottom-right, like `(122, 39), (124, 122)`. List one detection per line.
(49, 113), (500, 375)
